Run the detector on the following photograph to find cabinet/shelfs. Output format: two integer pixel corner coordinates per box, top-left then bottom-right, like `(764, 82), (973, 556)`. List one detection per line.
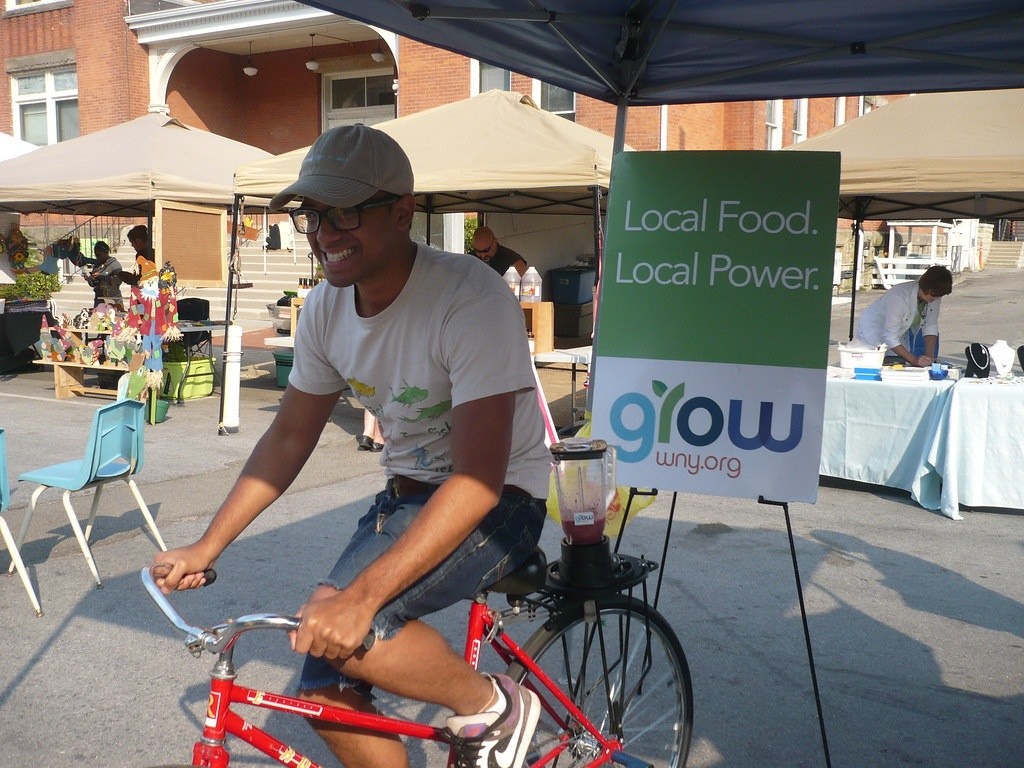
(518, 302), (554, 354)
(32, 324), (152, 402)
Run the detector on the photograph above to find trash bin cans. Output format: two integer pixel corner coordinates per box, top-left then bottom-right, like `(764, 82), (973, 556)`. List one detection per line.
(272, 352), (295, 388)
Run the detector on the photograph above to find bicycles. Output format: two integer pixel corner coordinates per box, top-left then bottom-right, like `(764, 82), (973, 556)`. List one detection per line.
(140, 550), (695, 768)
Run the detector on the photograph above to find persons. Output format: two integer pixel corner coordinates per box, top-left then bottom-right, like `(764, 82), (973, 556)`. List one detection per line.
(466, 226), (527, 276)
(358, 408), (385, 452)
(156, 124), (553, 768)
(857, 266), (953, 368)
(119, 225), (155, 287)
(80, 241), (123, 308)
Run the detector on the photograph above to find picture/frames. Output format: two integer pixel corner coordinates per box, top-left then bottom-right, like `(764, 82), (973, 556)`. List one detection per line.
(155, 198), (230, 290)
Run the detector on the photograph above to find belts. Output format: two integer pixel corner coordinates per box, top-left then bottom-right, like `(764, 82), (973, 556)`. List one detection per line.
(392, 474), (531, 498)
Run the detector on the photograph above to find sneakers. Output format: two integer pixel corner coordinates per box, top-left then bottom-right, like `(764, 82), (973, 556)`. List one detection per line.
(444, 673), (541, 768)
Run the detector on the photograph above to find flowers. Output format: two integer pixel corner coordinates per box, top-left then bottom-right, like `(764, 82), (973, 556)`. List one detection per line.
(0, 228), (30, 266)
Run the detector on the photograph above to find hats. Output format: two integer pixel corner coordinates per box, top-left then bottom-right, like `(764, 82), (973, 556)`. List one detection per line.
(269, 123), (414, 208)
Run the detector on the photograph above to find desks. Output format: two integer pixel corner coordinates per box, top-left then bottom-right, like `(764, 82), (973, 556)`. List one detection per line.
(819, 365), (959, 513)
(950, 376), (1024, 511)
(178, 325), (226, 405)
(533, 346), (593, 435)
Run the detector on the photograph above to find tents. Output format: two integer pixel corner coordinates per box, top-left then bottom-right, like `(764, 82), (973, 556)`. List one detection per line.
(218, 88), (639, 436)
(0, 112), (302, 258)
(299, 0), (1024, 408)
(779, 88), (1024, 342)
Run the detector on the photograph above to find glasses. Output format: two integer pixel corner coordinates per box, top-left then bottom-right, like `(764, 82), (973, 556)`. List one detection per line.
(289, 197), (399, 234)
(475, 239), (494, 253)
(928, 289), (942, 297)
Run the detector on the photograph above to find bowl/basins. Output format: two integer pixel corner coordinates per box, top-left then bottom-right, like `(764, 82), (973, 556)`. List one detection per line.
(266, 304), (302, 330)
(929, 369), (949, 380)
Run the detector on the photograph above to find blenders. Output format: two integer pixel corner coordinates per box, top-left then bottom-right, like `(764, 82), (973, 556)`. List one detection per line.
(549, 438), (615, 576)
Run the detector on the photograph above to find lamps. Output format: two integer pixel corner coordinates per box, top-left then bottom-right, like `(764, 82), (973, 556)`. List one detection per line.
(304, 34), (321, 70)
(370, 34), (387, 62)
(243, 40), (259, 76)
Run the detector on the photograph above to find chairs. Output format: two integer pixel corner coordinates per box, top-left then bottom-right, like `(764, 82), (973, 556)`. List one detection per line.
(0, 427), (45, 620)
(8, 399), (168, 587)
(176, 298), (213, 358)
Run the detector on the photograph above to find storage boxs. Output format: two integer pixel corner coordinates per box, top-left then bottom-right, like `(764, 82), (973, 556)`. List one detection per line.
(272, 350), (294, 388)
(546, 265), (593, 304)
(837, 342), (887, 370)
(159, 352), (216, 403)
(554, 301), (593, 337)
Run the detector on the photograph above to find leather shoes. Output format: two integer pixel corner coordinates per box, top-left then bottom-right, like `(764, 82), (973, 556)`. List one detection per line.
(359, 435), (374, 449)
(370, 441), (384, 452)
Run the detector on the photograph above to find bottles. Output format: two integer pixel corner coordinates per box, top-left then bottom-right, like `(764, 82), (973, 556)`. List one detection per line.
(296, 278), (324, 299)
(527, 329), (535, 356)
(502, 263), (520, 301)
(521, 265), (542, 303)
